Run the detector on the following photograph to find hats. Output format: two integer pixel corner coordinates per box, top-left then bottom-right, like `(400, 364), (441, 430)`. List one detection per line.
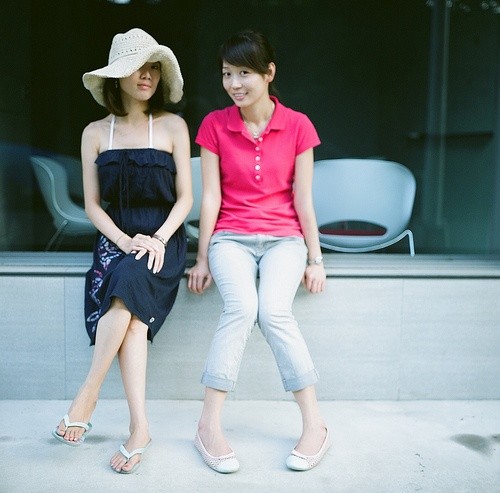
(82, 27), (184, 106)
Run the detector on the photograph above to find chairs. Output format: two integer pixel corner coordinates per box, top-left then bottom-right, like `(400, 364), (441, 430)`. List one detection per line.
(310, 159), (415, 255)
(25, 154), (202, 253)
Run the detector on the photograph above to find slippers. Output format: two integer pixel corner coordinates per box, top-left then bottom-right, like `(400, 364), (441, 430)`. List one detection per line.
(51, 413), (93, 447)
(116, 438), (152, 474)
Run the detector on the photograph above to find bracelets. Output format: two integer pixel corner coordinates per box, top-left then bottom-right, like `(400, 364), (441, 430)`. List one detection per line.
(115, 233), (126, 245)
(306, 256), (324, 266)
(152, 233), (167, 245)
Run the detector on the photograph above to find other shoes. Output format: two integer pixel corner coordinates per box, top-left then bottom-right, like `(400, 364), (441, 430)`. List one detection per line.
(286, 425), (333, 471)
(194, 432), (240, 474)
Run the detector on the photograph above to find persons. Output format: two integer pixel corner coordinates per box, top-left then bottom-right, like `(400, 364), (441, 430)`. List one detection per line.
(51, 28), (193, 475)
(186, 29), (331, 475)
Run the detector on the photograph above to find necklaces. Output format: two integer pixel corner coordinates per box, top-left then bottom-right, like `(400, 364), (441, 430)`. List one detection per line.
(240, 100), (275, 138)
